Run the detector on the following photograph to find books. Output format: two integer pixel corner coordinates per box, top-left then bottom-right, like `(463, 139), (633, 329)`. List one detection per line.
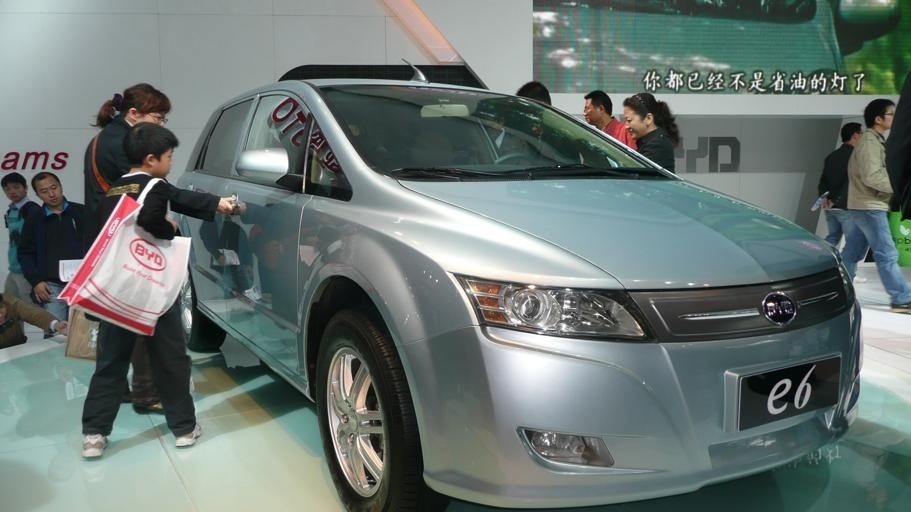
(810, 191), (829, 212)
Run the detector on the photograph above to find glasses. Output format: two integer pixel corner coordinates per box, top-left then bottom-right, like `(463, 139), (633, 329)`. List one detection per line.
(149, 113), (168, 123)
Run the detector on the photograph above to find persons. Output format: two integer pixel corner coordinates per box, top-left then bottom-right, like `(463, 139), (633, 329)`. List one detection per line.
(84, 84), (170, 414)
(517, 81), (551, 104)
(623, 93), (678, 173)
(819, 71), (910, 312)
(0, 173), (41, 334)
(81, 121), (234, 456)
(21, 171), (85, 339)
(0, 292), (68, 348)
(467, 108), (542, 165)
(584, 90), (637, 151)
(200, 204), (356, 328)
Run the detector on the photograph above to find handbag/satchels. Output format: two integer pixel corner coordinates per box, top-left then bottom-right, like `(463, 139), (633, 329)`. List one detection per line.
(57, 192), (192, 336)
(64, 306), (100, 359)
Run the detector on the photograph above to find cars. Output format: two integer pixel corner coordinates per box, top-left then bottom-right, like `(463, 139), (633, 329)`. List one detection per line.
(170, 59), (862, 512)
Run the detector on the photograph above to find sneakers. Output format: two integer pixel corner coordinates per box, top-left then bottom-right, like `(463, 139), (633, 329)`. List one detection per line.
(892, 301), (911, 314)
(175, 423), (200, 448)
(83, 433), (108, 457)
(119, 391), (166, 415)
